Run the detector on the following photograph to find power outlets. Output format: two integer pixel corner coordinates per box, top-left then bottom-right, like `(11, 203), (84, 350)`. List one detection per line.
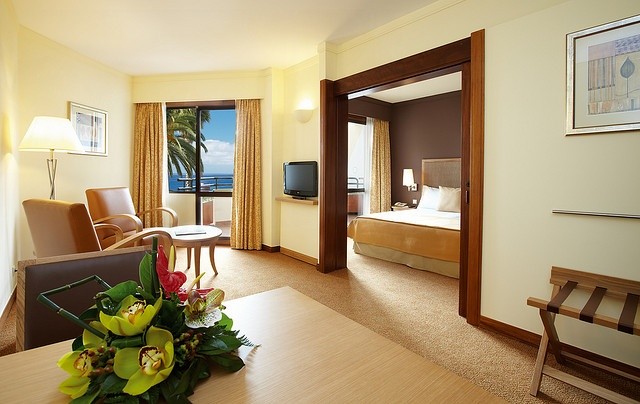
(10, 267), (16, 274)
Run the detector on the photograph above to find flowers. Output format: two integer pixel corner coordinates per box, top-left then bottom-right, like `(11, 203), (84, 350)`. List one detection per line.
(36, 236), (261, 404)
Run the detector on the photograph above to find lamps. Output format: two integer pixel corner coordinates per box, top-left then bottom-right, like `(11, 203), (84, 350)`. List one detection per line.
(402, 169), (417, 191)
(18, 116), (85, 201)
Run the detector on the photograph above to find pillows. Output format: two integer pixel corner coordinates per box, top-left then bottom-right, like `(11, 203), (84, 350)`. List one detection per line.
(416, 185), (439, 209)
(435, 185), (461, 212)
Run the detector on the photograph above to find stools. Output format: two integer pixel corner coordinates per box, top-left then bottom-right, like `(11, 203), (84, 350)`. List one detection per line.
(526, 265), (640, 403)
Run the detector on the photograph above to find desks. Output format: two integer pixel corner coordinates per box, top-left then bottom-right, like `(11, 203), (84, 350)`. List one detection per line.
(1, 284), (512, 404)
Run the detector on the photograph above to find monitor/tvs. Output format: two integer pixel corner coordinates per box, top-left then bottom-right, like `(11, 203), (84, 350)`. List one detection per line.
(283, 161), (319, 200)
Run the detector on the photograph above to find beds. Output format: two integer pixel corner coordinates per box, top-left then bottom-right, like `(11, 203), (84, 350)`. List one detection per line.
(347, 158), (462, 280)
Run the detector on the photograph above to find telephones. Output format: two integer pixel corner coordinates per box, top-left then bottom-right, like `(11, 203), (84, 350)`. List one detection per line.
(393, 202), (408, 206)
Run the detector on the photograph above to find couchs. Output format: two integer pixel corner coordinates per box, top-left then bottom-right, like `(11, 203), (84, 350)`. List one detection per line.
(86, 187), (178, 250)
(21, 198), (175, 272)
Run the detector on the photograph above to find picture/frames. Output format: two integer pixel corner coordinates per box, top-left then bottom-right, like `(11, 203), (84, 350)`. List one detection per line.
(565, 14), (640, 135)
(67, 101), (108, 157)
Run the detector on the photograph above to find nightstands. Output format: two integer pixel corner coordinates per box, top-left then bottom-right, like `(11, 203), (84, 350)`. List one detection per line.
(391, 206), (409, 211)
